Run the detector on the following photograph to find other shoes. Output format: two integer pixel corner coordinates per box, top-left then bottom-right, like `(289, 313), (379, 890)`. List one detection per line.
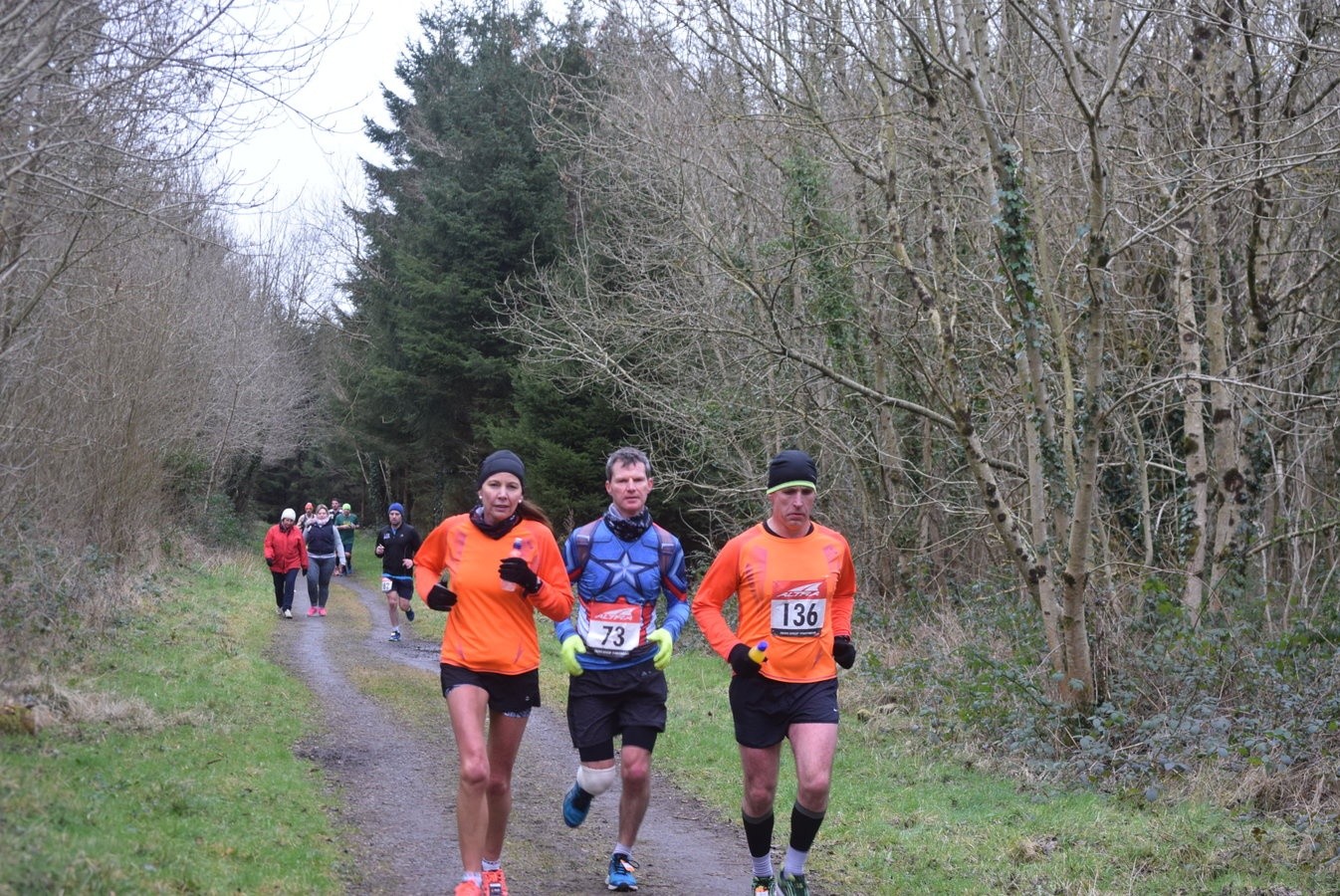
(348, 565), (352, 573)
(335, 567), (340, 575)
(341, 570), (346, 576)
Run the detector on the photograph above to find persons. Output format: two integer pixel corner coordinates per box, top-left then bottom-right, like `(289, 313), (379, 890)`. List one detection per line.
(693, 449), (857, 896)
(555, 447), (690, 892)
(412, 450), (573, 895)
(263, 498), (361, 618)
(374, 503), (422, 641)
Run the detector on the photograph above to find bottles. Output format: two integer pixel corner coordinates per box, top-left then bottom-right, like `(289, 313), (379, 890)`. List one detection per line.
(748, 641), (768, 664)
(501, 537), (522, 592)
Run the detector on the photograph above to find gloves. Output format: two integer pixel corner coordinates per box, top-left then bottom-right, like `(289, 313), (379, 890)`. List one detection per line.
(498, 556), (542, 593)
(833, 637), (857, 669)
(728, 644), (767, 676)
(427, 579), (461, 611)
(302, 568), (309, 576)
(561, 634), (586, 676)
(644, 628), (673, 669)
(266, 558), (273, 567)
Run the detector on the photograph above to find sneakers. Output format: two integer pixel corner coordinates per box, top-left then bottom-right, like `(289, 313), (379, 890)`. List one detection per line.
(390, 631), (400, 642)
(405, 608), (414, 621)
(454, 876), (483, 896)
(276, 604), (284, 614)
(307, 607), (318, 616)
(775, 862), (809, 896)
(605, 852), (640, 891)
(481, 866), (509, 896)
(750, 872), (777, 896)
(562, 782), (595, 827)
(284, 608), (292, 618)
(318, 608), (328, 616)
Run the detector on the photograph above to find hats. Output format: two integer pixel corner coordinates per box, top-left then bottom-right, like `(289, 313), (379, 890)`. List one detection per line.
(478, 449), (523, 489)
(389, 503), (406, 517)
(281, 508), (296, 522)
(343, 504), (351, 510)
(767, 450), (817, 498)
